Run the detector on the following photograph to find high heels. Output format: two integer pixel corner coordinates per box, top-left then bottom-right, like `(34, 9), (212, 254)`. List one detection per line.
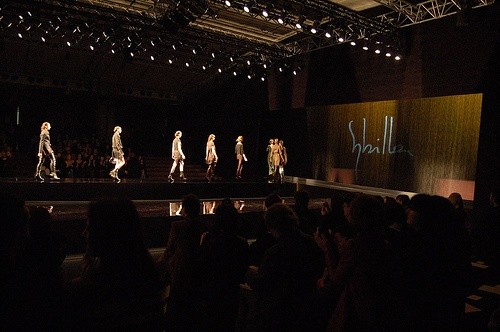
(36, 175), (44, 182)
(50, 174), (60, 180)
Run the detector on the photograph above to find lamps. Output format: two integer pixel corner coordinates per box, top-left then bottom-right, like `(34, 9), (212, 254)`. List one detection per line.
(0, 0), (404, 81)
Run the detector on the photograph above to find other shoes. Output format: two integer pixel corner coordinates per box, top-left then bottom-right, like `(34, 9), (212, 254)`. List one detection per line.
(180, 174), (187, 182)
(167, 176), (174, 182)
(116, 177), (120, 181)
(109, 172), (115, 178)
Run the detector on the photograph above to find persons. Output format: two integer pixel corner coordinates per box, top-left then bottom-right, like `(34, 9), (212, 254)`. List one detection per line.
(0, 122), (287, 185)
(0, 189), (500, 332)
